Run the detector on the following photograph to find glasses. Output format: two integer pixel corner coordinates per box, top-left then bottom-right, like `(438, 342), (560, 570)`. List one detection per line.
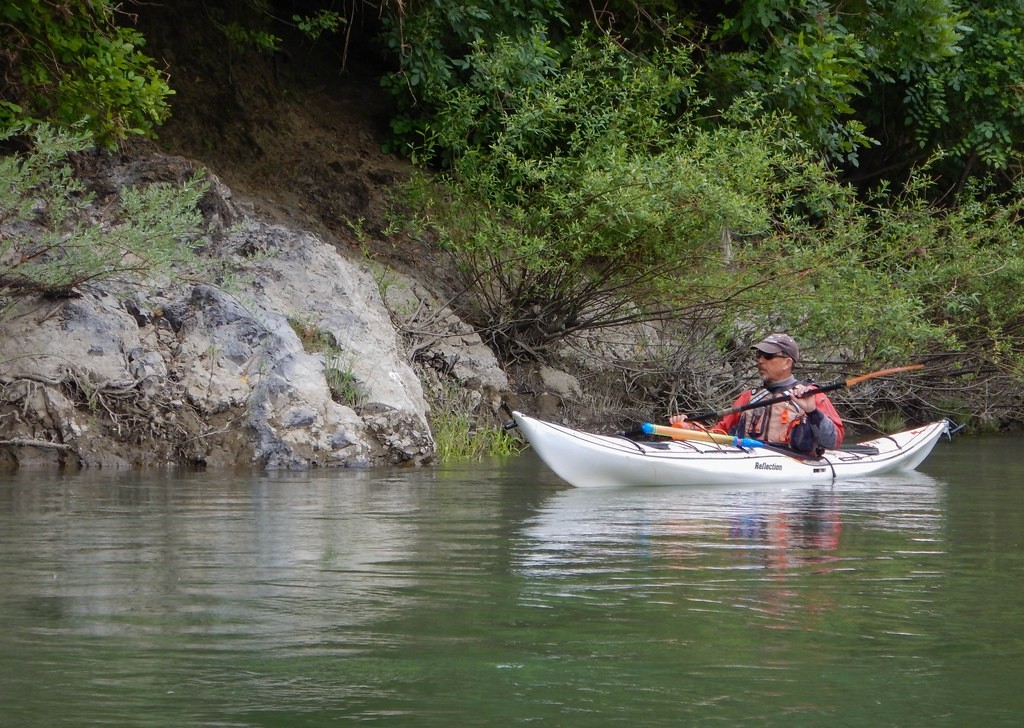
(755, 350), (786, 360)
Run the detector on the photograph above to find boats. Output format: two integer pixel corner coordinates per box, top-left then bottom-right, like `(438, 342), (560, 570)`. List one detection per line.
(512, 410), (950, 488)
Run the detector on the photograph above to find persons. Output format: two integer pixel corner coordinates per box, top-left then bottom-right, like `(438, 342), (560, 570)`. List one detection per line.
(669, 333), (844, 457)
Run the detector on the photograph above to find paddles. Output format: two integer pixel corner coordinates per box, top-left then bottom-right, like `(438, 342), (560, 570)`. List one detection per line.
(614, 361), (926, 437)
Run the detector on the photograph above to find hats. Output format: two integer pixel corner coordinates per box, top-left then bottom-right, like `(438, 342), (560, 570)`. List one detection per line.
(750, 333), (799, 363)
